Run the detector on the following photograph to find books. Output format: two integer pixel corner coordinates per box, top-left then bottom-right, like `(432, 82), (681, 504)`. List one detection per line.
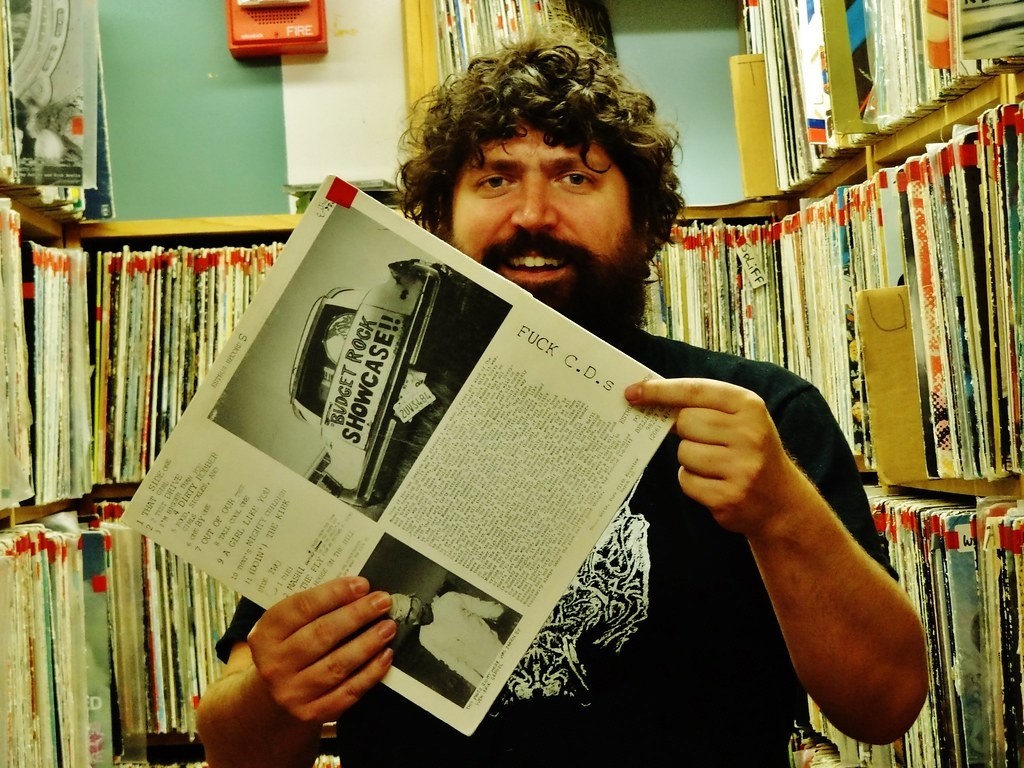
(0, 0), (1023, 768)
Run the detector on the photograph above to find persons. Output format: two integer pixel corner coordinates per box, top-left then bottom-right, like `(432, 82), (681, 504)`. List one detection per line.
(385, 591), (504, 688)
(196, 23), (930, 768)
